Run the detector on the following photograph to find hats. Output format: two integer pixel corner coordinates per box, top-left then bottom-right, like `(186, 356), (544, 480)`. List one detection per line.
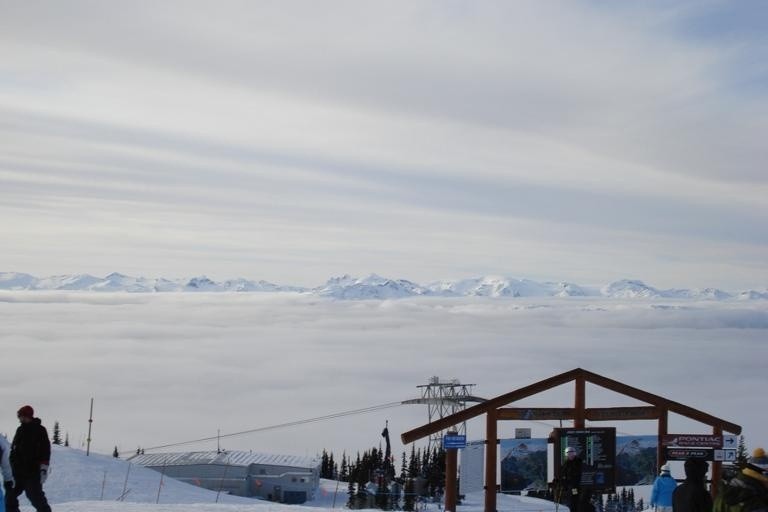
(18, 406), (33, 418)
(661, 465), (671, 472)
(753, 448), (766, 458)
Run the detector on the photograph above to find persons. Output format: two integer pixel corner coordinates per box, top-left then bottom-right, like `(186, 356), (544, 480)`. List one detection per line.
(4, 404), (52, 511)
(0, 433), (16, 511)
(557, 434), (598, 512)
(648, 463), (678, 511)
(711, 447), (768, 511)
(670, 456), (713, 511)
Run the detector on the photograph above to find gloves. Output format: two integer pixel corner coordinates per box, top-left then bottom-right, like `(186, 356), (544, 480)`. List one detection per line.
(3, 477), (16, 490)
(40, 463), (49, 484)
